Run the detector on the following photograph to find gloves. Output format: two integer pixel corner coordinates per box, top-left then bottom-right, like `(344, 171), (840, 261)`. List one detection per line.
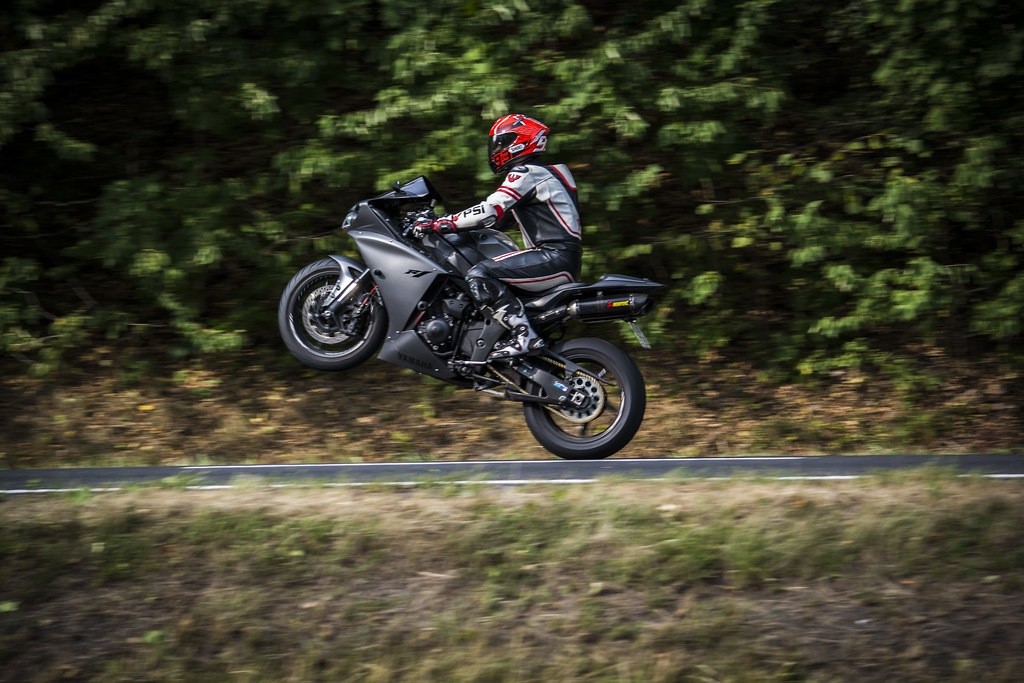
(412, 214), (460, 236)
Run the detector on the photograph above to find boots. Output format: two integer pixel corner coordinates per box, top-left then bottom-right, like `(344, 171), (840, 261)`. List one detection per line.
(487, 297), (545, 360)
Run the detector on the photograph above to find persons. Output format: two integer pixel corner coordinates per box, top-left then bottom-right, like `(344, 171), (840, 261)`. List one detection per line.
(413, 113), (581, 363)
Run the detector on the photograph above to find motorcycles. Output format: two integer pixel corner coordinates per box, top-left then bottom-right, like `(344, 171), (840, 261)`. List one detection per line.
(275, 173), (671, 461)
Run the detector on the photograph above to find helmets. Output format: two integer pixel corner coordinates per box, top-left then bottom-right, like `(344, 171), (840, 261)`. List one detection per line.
(488, 114), (550, 175)
